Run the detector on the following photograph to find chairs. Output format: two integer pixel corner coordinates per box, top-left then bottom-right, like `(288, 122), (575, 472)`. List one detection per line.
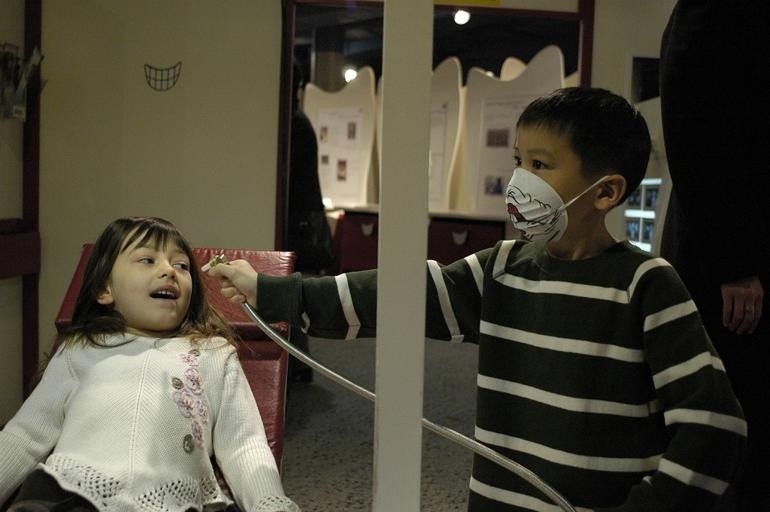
(53, 241), (299, 484)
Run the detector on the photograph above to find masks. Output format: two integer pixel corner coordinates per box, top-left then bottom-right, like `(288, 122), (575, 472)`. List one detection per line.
(505, 166), (610, 248)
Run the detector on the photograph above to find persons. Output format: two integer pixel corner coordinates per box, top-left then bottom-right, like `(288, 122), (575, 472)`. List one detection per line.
(284, 54), (336, 383)
(208, 86), (751, 510)
(0, 212), (298, 511)
(647, 1), (770, 510)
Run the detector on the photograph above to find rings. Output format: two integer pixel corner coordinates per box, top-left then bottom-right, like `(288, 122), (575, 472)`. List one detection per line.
(743, 304), (756, 314)
